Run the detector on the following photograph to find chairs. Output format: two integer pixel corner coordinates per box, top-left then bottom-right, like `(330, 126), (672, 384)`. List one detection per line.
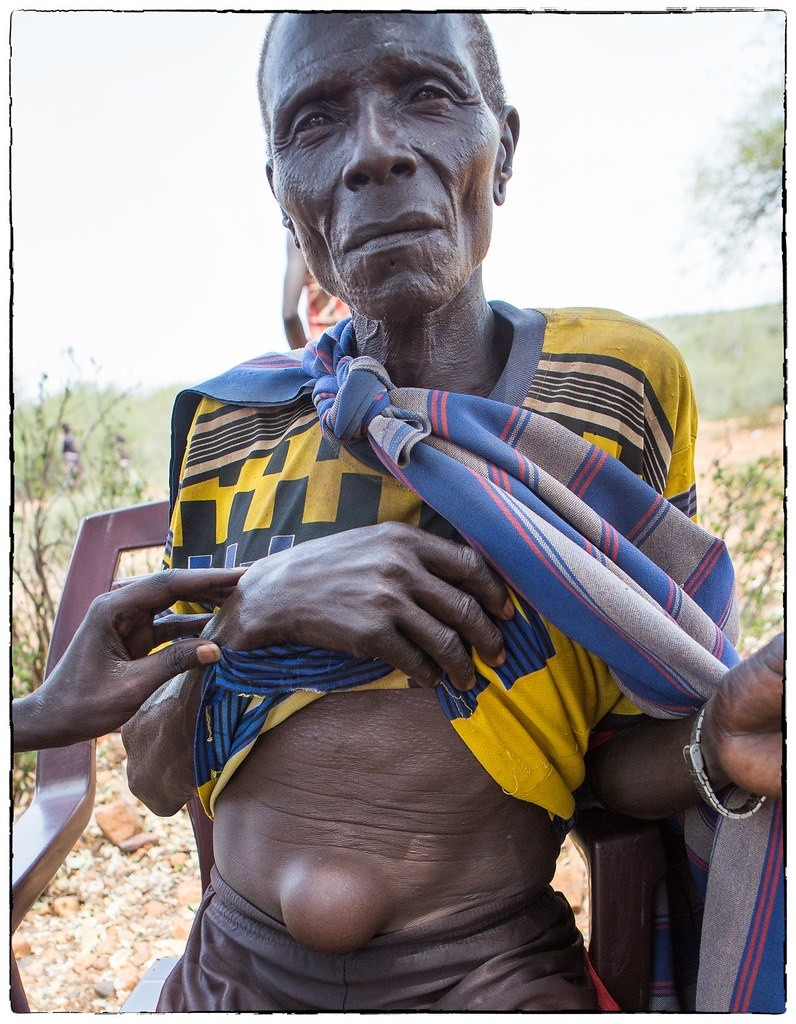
(10, 499), (663, 1013)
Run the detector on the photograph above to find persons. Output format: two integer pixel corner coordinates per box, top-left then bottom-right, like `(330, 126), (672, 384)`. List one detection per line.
(12, 567), (252, 751)
(121, 13), (787, 1016)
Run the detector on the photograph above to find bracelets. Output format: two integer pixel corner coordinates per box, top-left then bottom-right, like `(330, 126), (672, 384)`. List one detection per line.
(683, 701), (765, 819)
